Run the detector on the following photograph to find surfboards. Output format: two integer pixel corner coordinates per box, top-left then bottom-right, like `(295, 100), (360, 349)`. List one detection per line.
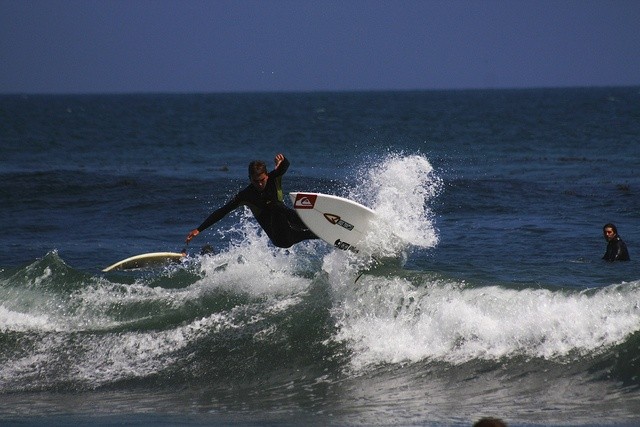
(99, 251), (192, 273)
(287, 192), (377, 251)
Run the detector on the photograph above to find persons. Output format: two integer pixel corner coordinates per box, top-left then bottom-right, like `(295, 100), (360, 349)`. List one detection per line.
(603, 216), (631, 263)
(185, 153), (319, 247)
